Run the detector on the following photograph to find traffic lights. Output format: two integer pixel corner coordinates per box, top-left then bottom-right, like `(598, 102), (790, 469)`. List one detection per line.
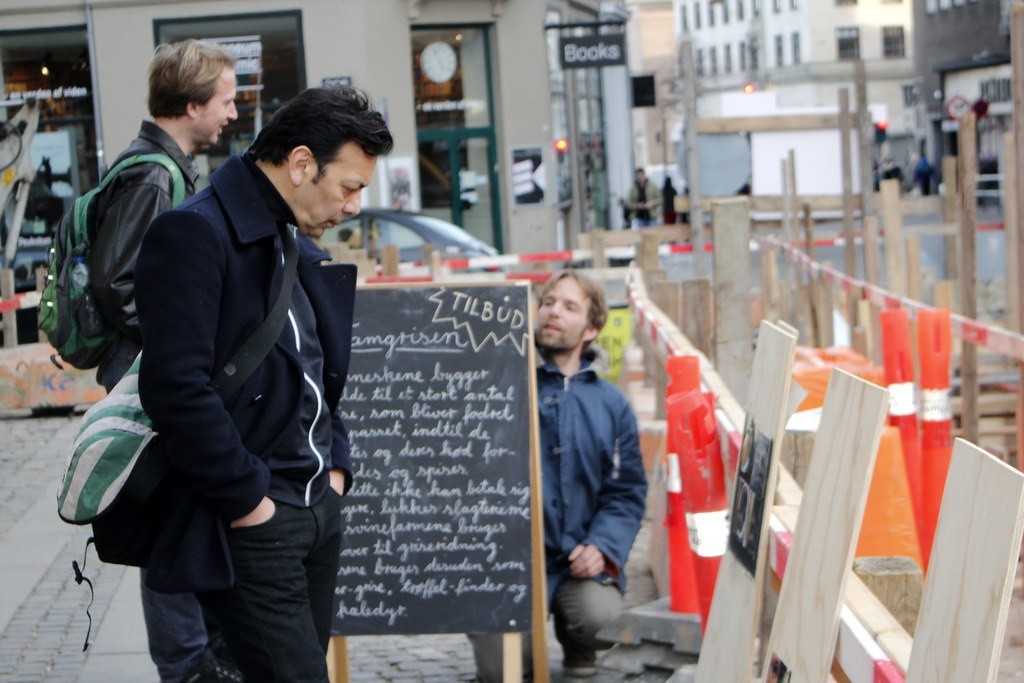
(875, 121), (889, 144)
(743, 83), (754, 95)
(554, 140), (568, 165)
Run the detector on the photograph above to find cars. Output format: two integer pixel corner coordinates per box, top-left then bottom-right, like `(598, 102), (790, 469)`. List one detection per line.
(323, 206), (498, 275)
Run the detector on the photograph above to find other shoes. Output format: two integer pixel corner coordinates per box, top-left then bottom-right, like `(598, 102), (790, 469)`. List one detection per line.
(563, 643), (597, 677)
(176, 649), (245, 683)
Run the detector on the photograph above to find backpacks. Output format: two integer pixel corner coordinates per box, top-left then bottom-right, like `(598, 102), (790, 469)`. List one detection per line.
(38, 153), (185, 370)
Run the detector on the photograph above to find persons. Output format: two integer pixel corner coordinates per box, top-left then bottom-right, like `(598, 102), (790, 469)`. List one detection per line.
(466, 269), (649, 683)
(133, 87), (394, 683)
(867, 140), (940, 196)
(84, 39), (249, 683)
(661, 177), (678, 224)
(737, 179), (751, 196)
(621, 168), (663, 230)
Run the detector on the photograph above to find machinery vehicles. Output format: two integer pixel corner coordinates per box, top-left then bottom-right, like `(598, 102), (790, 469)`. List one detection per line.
(0, 95), (44, 279)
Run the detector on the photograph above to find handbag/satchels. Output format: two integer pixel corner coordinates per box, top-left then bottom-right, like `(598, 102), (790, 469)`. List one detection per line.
(56, 349), (160, 567)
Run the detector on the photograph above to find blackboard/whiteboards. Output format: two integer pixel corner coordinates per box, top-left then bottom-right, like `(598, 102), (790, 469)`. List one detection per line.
(327, 276), (534, 637)
